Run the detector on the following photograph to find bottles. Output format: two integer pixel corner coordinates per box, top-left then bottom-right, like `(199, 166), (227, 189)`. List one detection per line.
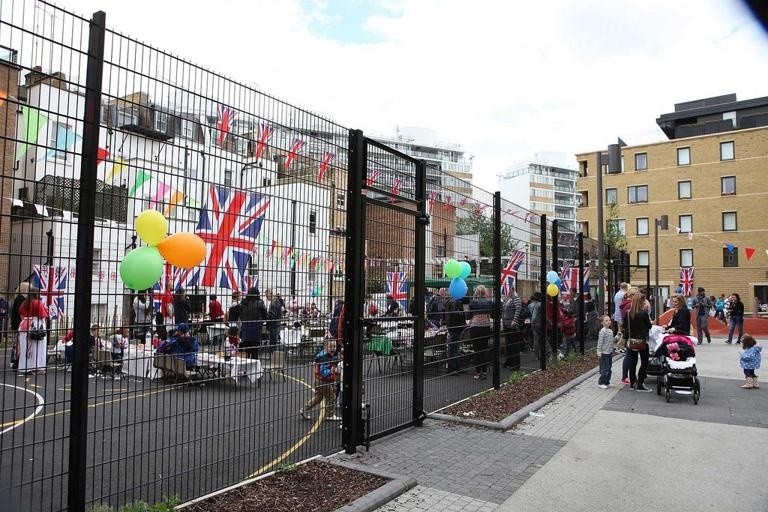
(145, 331), (158, 351)
(224, 337), (231, 361)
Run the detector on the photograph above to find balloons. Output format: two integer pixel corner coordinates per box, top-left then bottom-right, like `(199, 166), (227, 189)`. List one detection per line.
(444, 259), (471, 300)
(546, 271), (561, 297)
(121, 208), (207, 291)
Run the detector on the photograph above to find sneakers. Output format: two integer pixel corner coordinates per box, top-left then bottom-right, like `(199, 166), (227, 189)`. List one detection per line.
(326, 415), (342, 421)
(597, 382), (614, 389)
(620, 377), (652, 392)
(725, 339), (732, 343)
(299, 408), (311, 420)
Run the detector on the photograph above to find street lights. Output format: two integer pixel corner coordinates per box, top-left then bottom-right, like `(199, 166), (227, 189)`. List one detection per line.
(655, 216), (669, 324)
(596, 144), (621, 331)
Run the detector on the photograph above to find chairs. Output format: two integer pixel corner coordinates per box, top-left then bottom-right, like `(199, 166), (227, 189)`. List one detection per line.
(48, 319), (490, 393)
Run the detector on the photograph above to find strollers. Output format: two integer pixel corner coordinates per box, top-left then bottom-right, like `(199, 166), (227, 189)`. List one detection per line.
(647, 329), (700, 406)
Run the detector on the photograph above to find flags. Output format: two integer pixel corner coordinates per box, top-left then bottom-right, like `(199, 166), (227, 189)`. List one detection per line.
(385, 272), (408, 312)
(170, 183), (271, 292)
(32, 264), (67, 320)
(501, 250), (525, 295)
(678, 269), (694, 297)
(561, 268), (590, 295)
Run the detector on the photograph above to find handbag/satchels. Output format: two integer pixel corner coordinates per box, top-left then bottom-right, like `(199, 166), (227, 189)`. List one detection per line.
(627, 338), (647, 352)
(30, 324), (46, 340)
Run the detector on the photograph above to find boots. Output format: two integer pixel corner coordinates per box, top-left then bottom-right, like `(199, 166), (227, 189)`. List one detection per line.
(741, 375), (759, 389)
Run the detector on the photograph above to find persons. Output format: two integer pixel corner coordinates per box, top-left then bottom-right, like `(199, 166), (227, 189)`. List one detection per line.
(300, 338), (343, 420)
(737, 334), (762, 388)
(692, 287), (744, 344)
(329, 300), (370, 409)
(132, 288), (322, 370)
(367, 286), (601, 379)
(596, 315), (614, 388)
(1, 282), (47, 375)
(615, 282), (691, 391)
(63, 325), (129, 380)
